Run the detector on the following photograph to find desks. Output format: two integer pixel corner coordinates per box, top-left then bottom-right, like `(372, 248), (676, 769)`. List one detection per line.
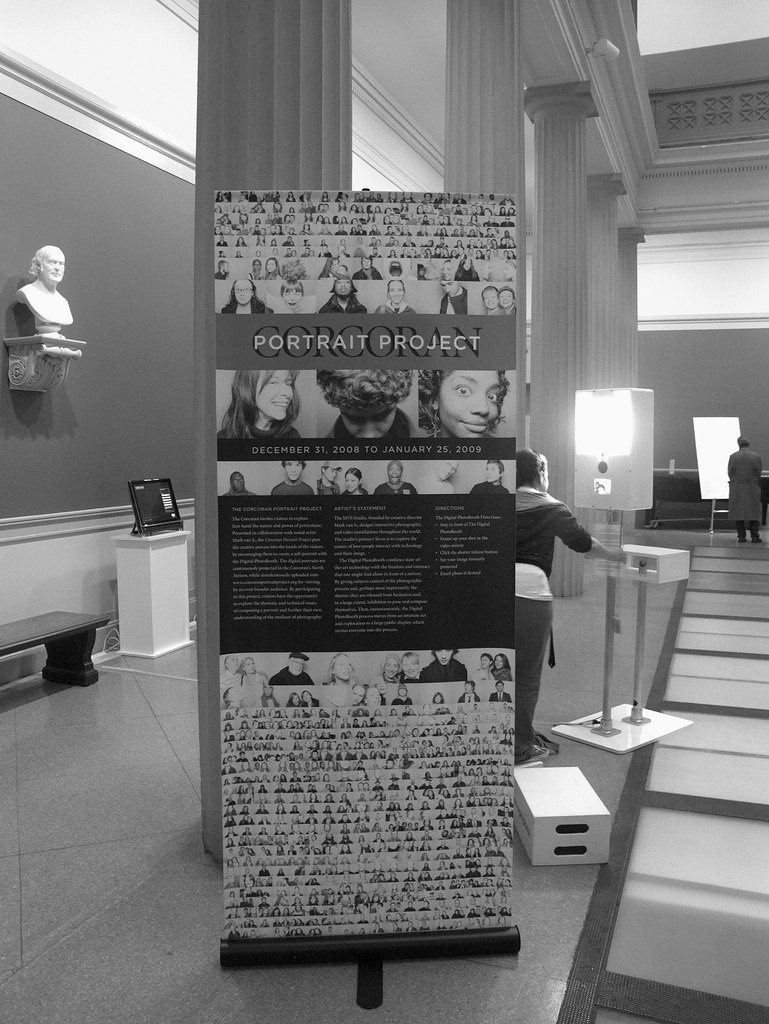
(645, 467), (769, 528)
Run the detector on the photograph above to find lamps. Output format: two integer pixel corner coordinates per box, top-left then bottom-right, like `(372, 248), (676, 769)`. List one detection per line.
(586, 37), (620, 62)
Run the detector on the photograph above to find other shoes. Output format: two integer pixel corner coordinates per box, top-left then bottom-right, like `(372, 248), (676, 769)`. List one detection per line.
(738, 538), (747, 542)
(752, 538), (762, 543)
(515, 744), (549, 764)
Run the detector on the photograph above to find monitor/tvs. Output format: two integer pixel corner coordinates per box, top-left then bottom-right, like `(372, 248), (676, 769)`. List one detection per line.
(128, 478), (183, 536)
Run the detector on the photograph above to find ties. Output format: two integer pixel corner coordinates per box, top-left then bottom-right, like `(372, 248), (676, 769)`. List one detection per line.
(468, 697), (471, 702)
(499, 693), (501, 701)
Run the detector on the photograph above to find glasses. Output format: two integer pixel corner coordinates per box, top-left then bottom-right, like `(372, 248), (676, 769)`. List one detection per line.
(234, 287), (251, 295)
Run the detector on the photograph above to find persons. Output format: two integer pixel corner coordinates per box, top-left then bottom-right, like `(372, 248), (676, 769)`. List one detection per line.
(221, 681), (514, 940)
(322, 653), (356, 684)
(217, 370), (302, 438)
(316, 370), (417, 439)
(418, 370), (510, 438)
(16, 245), (73, 339)
(728, 435), (762, 543)
(374, 649), (514, 683)
(221, 655), (269, 685)
(214, 191), (516, 315)
(221, 460), (512, 496)
(269, 652), (315, 685)
(514, 448), (627, 765)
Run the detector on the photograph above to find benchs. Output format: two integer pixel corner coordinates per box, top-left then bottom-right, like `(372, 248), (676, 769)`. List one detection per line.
(0, 611), (110, 686)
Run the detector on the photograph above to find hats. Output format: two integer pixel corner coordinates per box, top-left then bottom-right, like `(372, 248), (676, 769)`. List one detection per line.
(390, 261), (402, 271)
(288, 652), (310, 663)
(397, 684), (407, 691)
(323, 461), (343, 472)
(498, 286), (515, 298)
